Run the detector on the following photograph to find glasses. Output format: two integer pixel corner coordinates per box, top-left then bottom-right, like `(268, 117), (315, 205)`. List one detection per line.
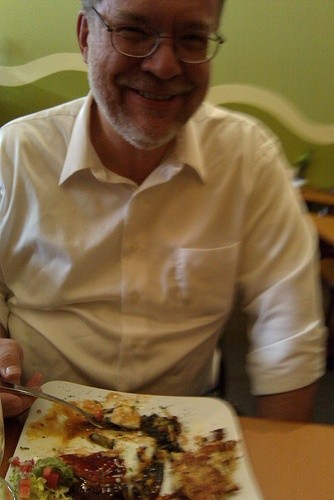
(90, 6), (224, 63)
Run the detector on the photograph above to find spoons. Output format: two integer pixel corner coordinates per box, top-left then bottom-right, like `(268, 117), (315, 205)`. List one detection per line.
(0, 382), (121, 431)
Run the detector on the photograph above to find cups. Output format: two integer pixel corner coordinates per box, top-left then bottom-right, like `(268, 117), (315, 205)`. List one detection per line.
(0, 476), (18, 500)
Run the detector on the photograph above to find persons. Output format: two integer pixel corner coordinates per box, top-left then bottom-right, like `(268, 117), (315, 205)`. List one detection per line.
(0, 0), (326, 419)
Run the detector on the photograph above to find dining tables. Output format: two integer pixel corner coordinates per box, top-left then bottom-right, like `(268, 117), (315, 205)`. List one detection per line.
(0, 414), (334, 500)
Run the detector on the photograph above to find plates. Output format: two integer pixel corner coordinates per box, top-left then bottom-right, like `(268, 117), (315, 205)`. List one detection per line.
(7, 381), (263, 500)
